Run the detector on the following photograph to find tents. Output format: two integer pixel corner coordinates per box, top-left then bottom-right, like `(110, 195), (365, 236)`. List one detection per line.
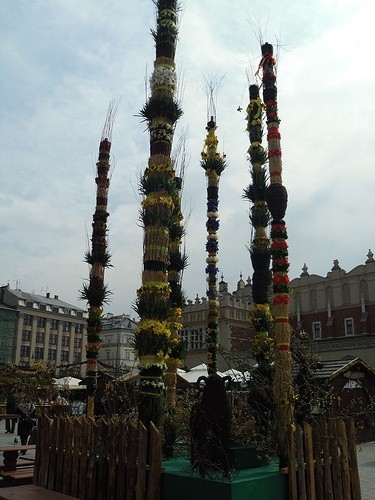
(37, 377), (90, 392)
(173, 362), (275, 383)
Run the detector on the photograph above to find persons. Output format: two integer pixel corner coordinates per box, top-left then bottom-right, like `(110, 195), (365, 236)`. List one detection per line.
(17, 395), (37, 456)
(94, 391), (107, 422)
(5, 385), (19, 432)
